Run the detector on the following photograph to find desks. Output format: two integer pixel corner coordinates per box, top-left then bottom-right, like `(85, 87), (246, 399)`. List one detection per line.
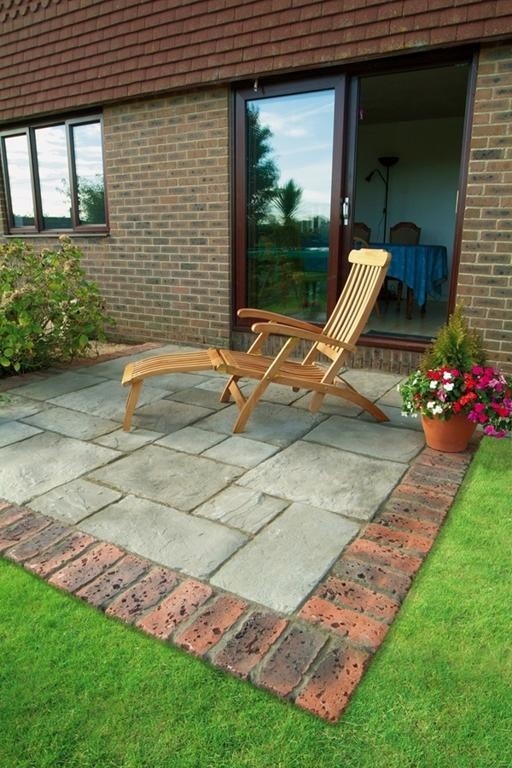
(281, 246), (449, 322)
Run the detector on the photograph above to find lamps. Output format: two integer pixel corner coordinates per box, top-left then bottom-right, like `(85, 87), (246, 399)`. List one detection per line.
(365, 155), (399, 244)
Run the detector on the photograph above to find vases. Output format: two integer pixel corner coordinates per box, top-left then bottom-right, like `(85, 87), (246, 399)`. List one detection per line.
(420, 405), (478, 453)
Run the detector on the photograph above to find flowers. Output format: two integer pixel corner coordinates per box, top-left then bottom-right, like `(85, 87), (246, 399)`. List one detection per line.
(397, 365), (512, 438)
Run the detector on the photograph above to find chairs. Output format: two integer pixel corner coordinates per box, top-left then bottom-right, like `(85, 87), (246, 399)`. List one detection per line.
(338, 221), (421, 319)
(120, 249), (392, 440)
